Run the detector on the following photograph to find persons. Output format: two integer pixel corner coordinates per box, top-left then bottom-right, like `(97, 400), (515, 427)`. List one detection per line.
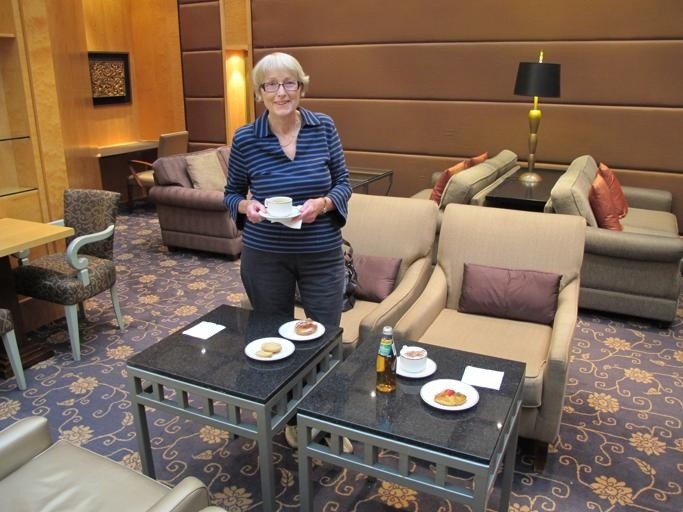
(221, 48), (356, 456)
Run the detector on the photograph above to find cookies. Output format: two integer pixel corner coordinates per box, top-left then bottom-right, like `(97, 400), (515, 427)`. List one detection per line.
(256, 341), (281, 358)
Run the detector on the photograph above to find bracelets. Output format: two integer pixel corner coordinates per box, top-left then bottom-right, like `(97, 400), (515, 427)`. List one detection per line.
(320, 196), (327, 216)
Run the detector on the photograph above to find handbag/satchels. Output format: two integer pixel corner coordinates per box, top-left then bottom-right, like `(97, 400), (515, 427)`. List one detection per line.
(293, 238), (356, 311)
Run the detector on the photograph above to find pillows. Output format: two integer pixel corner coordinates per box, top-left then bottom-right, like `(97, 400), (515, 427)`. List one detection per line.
(184, 153), (227, 192)
(353, 256), (402, 303)
(589, 162), (629, 231)
(429, 151), (489, 206)
(458, 263), (563, 327)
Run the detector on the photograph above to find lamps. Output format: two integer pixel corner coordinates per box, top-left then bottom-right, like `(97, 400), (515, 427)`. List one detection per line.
(514, 48), (560, 183)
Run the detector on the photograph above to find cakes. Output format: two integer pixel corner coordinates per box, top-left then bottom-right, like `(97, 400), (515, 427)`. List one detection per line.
(435, 389), (467, 407)
(294, 317), (316, 337)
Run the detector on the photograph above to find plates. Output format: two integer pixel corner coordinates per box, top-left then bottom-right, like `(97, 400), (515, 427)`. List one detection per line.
(243, 337), (296, 361)
(419, 378), (479, 412)
(258, 206), (301, 220)
(277, 320), (326, 342)
(390, 354), (437, 379)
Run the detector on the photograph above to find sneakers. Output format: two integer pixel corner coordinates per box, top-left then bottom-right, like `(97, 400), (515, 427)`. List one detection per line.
(283, 424), (298, 450)
(325, 436), (356, 455)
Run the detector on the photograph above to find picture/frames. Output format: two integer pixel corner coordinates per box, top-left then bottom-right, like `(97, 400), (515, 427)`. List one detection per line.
(88, 51), (131, 105)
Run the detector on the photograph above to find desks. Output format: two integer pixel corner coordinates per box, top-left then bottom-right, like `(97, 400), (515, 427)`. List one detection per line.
(485, 167), (567, 213)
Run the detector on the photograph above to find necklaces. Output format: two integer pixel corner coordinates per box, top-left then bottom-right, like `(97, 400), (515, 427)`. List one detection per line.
(279, 112), (300, 147)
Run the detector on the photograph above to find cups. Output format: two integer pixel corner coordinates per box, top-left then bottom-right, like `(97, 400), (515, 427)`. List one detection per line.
(263, 196), (293, 216)
(400, 344), (428, 373)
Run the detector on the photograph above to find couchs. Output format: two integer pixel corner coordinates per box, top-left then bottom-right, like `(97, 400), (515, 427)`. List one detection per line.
(543, 155), (683, 329)
(148, 146), (242, 261)
(410, 150), (523, 206)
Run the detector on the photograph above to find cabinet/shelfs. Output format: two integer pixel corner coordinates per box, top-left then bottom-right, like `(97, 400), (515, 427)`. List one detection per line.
(0, 0), (69, 340)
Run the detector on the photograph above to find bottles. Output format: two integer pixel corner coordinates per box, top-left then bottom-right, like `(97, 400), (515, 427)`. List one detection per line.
(375, 325), (396, 392)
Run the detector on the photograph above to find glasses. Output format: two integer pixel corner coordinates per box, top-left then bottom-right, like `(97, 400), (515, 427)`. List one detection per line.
(258, 79), (304, 93)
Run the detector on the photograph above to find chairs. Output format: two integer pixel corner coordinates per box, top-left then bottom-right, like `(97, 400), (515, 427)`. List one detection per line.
(12, 188), (124, 361)
(0, 309), (27, 391)
(240, 193), (437, 356)
(0, 416), (210, 512)
(199, 506), (227, 512)
(126, 131), (189, 214)
(392, 203), (585, 474)
